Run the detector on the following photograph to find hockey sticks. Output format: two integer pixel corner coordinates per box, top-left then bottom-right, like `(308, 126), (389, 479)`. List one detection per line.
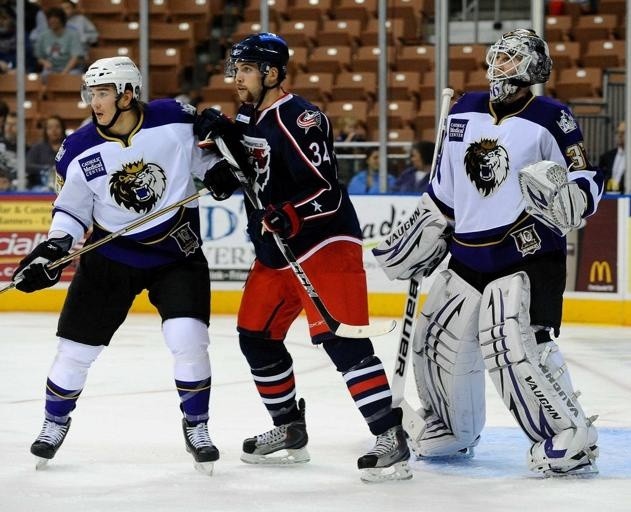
(212, 136), (397, 339)
(389, 88), (454, 442)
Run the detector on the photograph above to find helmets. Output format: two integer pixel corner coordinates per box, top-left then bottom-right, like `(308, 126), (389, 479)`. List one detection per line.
(230, 31), (290, 77)
(493, 27), (553, 87)
(82, 54), (143, 102)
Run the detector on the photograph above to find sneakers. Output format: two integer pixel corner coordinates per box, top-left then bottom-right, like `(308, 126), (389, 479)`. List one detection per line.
(356, 425), (409, 468)
(180, 417), (221, 461)
(31, 412), (73, 458)
(243, 412), (309, 454)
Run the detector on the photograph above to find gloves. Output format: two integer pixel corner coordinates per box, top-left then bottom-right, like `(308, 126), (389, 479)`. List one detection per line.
(252, 206), (290, 242)
(195, 108), (233, 144)
(204, 161), (242, 202)
(13, 239), (72, 289)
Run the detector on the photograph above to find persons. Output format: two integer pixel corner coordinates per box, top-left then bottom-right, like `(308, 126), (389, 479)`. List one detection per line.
(589, 120), (626, 195)
(193, 32), (415, 486)
(11, 56), (241, 476)
(0, 0), (99, 79)
(347, 147), (397, 194)
(1, 100), (69, 193)
(344, 118), (370, 154)
(391, 139), (435, 195)
(371, 26), (601, 481)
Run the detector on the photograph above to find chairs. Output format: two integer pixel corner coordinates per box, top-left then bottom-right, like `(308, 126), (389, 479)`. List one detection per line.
(0, 0), (628, 176)
